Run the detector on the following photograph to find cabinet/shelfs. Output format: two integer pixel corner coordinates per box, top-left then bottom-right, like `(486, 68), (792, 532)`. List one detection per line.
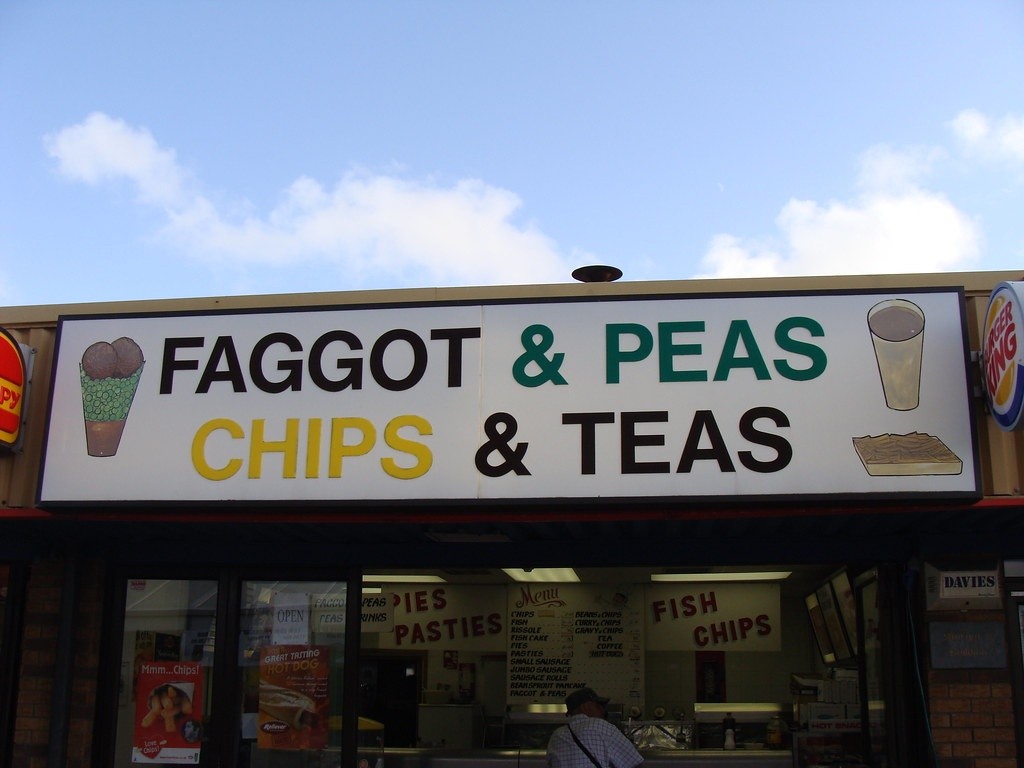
(418, 704), (486, 751)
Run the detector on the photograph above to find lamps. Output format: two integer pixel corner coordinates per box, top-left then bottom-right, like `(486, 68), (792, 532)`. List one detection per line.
(572, 266), (622, 282)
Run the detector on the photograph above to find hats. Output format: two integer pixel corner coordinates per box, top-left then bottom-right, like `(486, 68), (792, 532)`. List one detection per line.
(565, 687), (609, 715)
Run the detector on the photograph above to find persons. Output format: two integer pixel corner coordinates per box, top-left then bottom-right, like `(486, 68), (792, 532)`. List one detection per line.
(545, 689), (646, 768)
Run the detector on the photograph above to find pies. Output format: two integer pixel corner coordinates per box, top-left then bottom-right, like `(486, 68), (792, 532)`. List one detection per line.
(258, 680), (320, 732)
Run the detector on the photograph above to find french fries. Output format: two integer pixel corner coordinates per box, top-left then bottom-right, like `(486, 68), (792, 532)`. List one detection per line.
(140, 686), (192, 733)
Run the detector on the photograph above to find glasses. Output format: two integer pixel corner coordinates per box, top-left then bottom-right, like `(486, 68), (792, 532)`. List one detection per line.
(587, 703), (605, 708)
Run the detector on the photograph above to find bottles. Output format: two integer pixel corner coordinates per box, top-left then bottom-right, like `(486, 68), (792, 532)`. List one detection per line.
(766, 715), (783, 750)
(722, 712), (737, 751)
